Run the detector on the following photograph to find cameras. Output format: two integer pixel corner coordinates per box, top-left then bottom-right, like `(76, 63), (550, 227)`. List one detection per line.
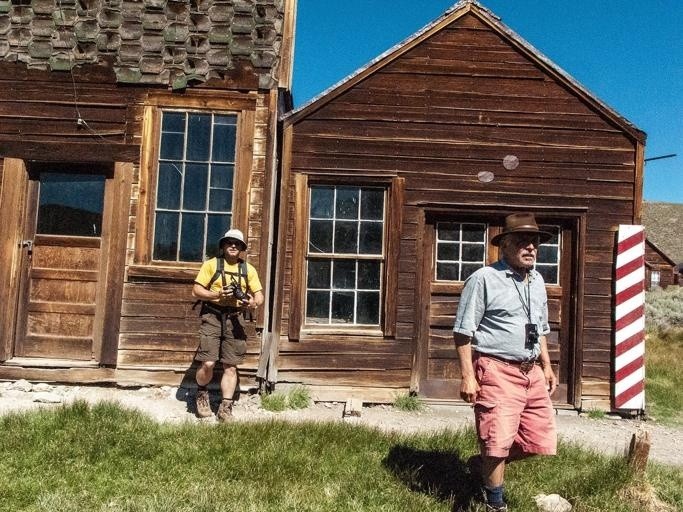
(523, 323), (540, 344)
(222, 282), (246, 302)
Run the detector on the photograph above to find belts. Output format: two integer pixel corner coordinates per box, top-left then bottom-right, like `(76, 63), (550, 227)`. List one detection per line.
(488, 354), (537, 371)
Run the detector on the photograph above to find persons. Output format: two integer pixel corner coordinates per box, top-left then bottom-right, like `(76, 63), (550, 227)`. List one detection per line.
(192, 228), (265, 424)
(453, 211), (558, 512)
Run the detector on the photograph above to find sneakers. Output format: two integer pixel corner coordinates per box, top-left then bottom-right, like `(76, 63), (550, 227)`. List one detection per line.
(466, 455), (507, 512)
(217, 399), (233, 421)
(196, 387), (212, 416)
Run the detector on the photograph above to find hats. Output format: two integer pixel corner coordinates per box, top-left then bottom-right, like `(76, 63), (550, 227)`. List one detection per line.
(219, 229), (247, 251)
(492, 213), (552, 246)
